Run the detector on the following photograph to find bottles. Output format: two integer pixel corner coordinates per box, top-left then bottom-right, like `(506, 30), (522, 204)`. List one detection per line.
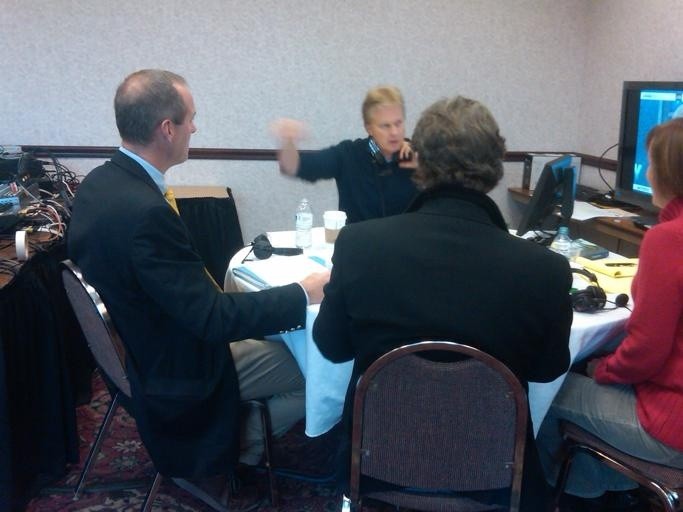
(548, 226), (572, 260)
(294, 198), (315, 251)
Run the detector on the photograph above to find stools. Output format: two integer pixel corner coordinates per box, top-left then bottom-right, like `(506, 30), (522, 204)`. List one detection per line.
(545, 415), (682, 511)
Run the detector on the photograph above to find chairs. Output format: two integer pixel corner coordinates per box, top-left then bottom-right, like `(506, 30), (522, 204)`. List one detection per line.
(59, 258), (281, 512)
(348, 337), (533, 511)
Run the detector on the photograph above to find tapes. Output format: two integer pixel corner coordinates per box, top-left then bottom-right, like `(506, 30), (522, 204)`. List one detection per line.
(15, 231), (29, 262)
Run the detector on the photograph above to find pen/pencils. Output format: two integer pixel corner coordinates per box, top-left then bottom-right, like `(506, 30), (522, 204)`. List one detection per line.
(605, 262), (637, 267)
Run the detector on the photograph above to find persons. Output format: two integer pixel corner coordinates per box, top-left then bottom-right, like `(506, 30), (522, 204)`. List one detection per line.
(312, 96), (574, 512)
(62, 68), (331, 509)
(532, 118), (682, 512)
(267, 86), (419, 222)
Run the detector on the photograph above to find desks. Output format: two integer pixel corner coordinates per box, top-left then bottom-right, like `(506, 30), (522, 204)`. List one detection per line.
(219, 222), (639, 457)
(0, 183), (85, 472)
(506, 181), (661, 260)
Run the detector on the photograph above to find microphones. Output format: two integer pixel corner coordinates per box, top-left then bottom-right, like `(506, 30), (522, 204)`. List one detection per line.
(601, 294), (629, 310)
(241, 247), (254, 264)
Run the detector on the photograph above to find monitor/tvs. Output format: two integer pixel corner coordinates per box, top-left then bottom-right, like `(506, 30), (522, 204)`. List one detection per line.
(613, 81), (683, 231)
(516, 154), (576, 246)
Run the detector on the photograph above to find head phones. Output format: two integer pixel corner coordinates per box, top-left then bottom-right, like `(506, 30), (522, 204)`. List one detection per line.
(253, 234), (303, 260)
(365, 136), (411, 167)
(570, 268), (606, 312)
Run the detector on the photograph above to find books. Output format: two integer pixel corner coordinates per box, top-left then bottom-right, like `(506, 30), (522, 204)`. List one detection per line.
(232, 250), (325, 290)
(576, 255), (639, 280)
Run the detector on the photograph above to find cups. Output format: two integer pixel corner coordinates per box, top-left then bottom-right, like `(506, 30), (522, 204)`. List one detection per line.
(323, 209), (349, 246)
